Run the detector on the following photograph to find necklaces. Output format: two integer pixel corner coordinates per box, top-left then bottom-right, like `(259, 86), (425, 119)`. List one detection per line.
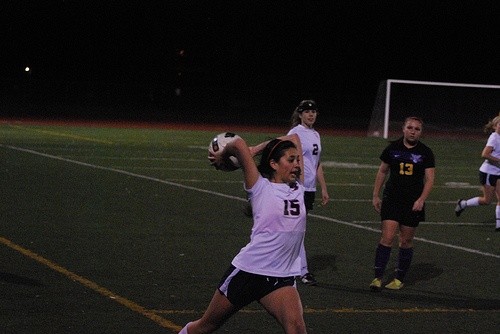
(401, 136), (417, 146)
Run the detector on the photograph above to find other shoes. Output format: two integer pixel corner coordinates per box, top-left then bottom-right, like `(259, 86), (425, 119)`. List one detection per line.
(455, 198), (464, 217)
(370, 277), (382, 289)
(385, 279), (404, 289)
(495, 227), (500, 231)
(301, 273), (317, 285)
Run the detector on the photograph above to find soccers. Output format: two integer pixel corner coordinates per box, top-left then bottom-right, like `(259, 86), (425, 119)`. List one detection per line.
(208, 132), (245, 171)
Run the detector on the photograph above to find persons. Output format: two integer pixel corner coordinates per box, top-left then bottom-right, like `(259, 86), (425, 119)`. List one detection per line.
(286, 100), (329, 285)
(369, 116), (435, 289)
(455, 115), (500, 232)
(178, 133), (307, 334)
